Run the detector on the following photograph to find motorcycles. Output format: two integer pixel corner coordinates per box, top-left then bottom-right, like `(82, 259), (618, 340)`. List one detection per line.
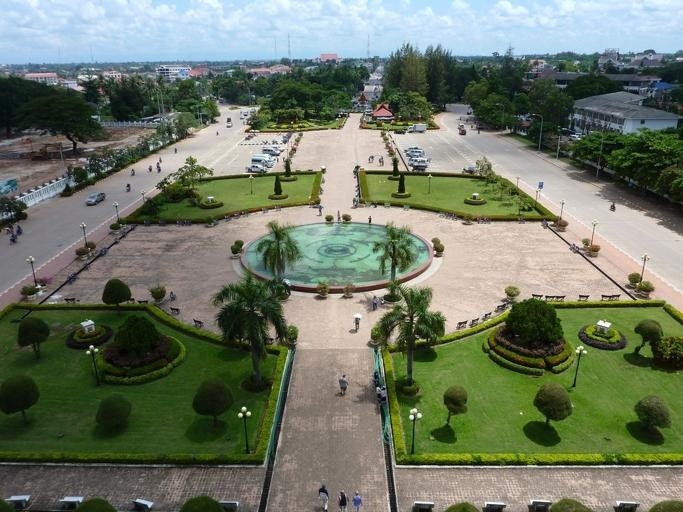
(126, 147), (178, 191)
(6, 225), (23, 245)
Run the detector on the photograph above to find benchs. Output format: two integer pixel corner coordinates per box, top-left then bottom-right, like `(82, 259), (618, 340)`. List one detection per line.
(530, 499), (552, 512)
(132, 498), (154, 509)
(60, 496), (84, 509)
(3, 495), (31, 508)
(219, 500), (240, 510)
(414, 501), (435, 510)
(616, 500), (640, 511)
(484, 501), (506, 511)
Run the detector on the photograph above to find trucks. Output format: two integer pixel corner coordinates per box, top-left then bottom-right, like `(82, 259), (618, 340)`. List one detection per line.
(408, 124), (426, 133)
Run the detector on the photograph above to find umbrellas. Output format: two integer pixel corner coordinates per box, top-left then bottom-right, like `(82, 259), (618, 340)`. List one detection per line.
(353, 313), (362, 318)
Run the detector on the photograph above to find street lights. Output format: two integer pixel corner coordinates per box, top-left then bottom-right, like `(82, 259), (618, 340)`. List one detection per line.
(496, 103), (505, 133)
(534, 188), (650, 282)
(408, 408), (421, 454)
(572, 346), (588, 387)
(26, 201), (122, 287)
(238, 405), (251, 454)
(427, 174), (433, 194)
(85, 345), (101, 386)
(532, 114), (543, 151)
(589, 131), (603, 177)
(248, 175), (255, 195)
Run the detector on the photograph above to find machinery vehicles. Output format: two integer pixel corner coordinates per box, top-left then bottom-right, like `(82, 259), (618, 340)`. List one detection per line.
(31, 142), (62, 161)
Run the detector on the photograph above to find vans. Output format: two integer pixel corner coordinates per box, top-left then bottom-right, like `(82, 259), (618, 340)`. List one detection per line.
(404, 146), (429, 170)
(247, 144), (285, 173)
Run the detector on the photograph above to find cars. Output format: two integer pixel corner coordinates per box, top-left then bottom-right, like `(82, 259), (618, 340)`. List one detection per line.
(459, 128), (467, 135)
(85, 191), (105, 206)
(569, 131), (584, 142)
(227, 107), (256, 127)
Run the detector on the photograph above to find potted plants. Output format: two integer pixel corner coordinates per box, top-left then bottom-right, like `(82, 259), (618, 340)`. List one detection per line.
(505, 286), (520, 301)
(21, 274), (50, 301)
(110, 218), (127, 235)
(557, 219), (568, 231)
(628, 272), (655, 298)
(230, 240), (244, 259)
(434, 244), (444, 256)
(582, 238), (601, 257)
(76, 241), (97, 260)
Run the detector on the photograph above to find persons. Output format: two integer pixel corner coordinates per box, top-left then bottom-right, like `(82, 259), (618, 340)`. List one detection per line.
(339, 490), (350, 512)
(131, 169), (135, 175)
(169, 290), (176, 301)
(368, 216), (372, 223)
(352, 490), (363, 512)
(355, 318), (360, 332)
(216, 131), (219, 136)
(148, 157), (162, 173)
(613, 200), (617, 209)
(372, 296), (379, 310)
(376, 385), (386, 402)
(7, 223), (23, 244)
(175, 148), (177, 152)
(339, 374), (349, 395)
(319, 205), (322, 215)
(126, 184), (131, 191)
(371, 371), (379, 384)
(319, 485), (329, 512)
